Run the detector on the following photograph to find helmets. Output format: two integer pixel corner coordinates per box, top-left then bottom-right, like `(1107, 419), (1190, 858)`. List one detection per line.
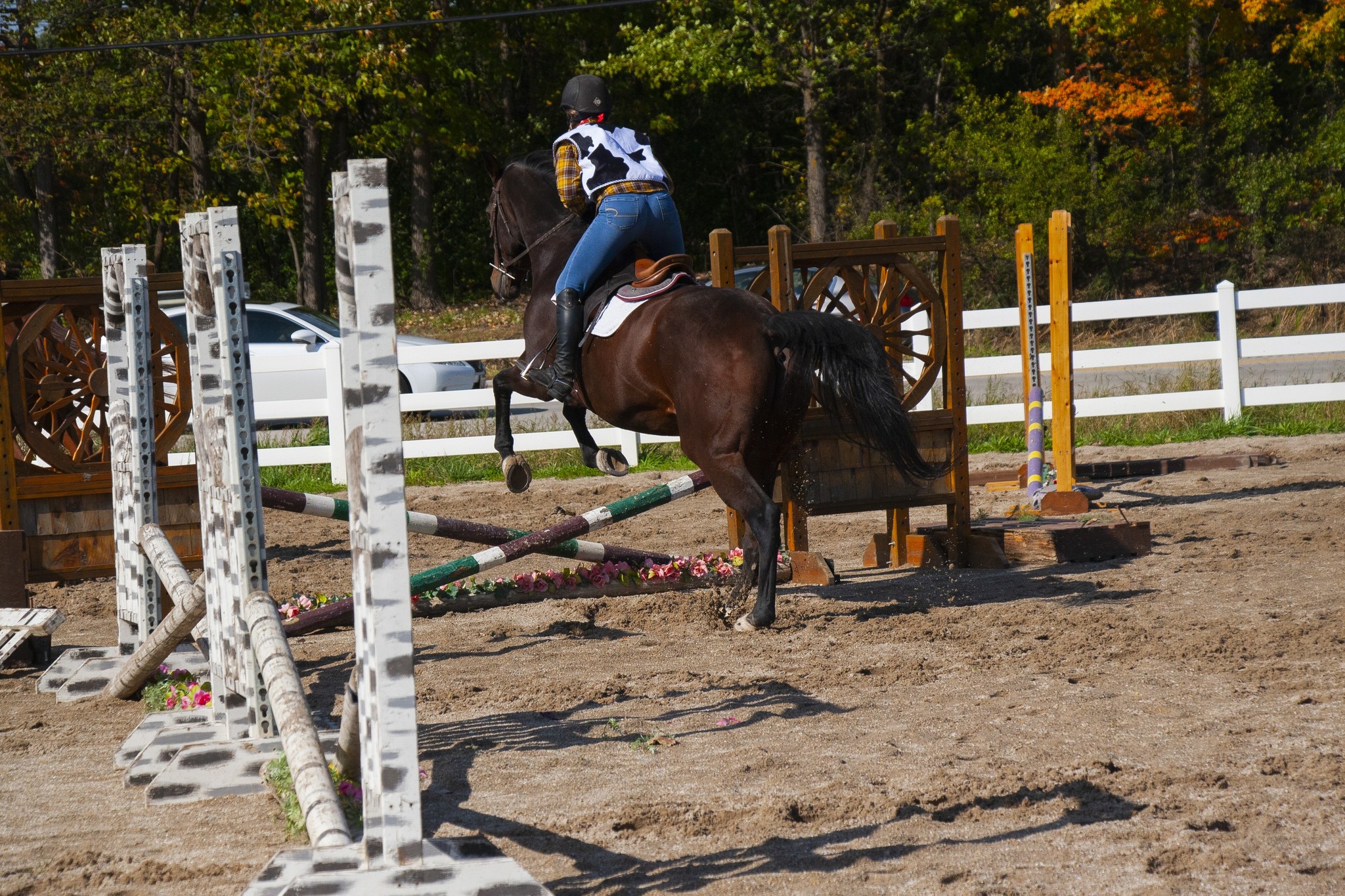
(560, 74), (611, 114)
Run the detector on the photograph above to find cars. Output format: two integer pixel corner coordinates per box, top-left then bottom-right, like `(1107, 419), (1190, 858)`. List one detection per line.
(696, 263), (924, 316)
(84, 281), (487, 393)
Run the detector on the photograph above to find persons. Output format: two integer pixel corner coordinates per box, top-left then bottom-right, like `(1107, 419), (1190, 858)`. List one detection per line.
(514, 75), (687, 404)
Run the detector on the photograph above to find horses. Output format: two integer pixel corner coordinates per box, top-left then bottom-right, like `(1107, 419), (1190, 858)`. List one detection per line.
(488, 161), (965, 633)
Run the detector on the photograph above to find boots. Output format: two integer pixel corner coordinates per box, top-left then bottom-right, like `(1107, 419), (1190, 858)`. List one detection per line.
(516, 287), (584, 403)
